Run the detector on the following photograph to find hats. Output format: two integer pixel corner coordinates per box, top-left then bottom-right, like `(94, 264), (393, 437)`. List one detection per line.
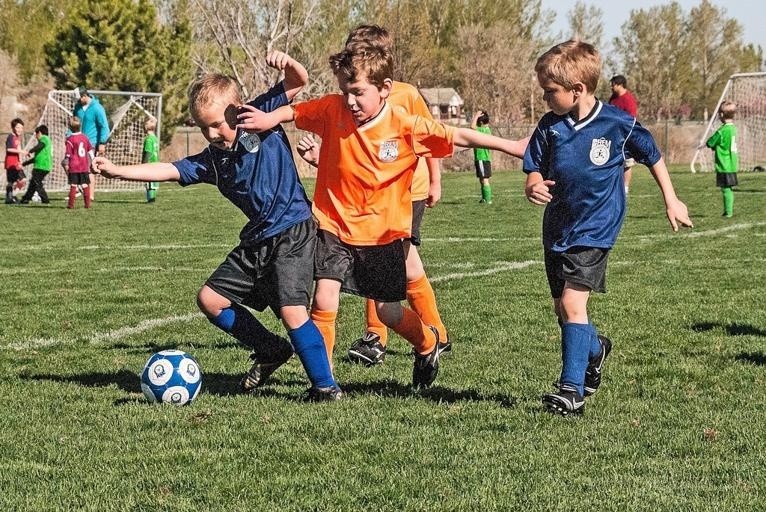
(71, 86), (86, 103)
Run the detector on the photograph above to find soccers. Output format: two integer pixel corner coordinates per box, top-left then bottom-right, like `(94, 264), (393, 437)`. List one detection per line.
(141, 349), (203, 407)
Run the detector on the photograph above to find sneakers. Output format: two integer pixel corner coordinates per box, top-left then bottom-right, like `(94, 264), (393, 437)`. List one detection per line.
(542, 391), (587, 416)
(297, 385), (346, 405)
(350, 331), (387, 365)
(239, 334), (294, 390)
(411, 326), (440, 388)
(584, 334), (612, 397)
(439, 332), (452, 356)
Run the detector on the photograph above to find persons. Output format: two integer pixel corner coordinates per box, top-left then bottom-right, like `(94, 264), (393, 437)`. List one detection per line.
(706, 100), (741, 219)
(520, 41), (693, 415)
(90, 49), (347, 400)
(4, 117), (31, 204)
(141, 116), (160, 202)
(470, 110), (494, 205)
(19, 125), (53, 203)
(606, 74), (638, 197)
(62, 116), (96, 209)
(65, 85), (110, 198)
(234, 42), (529, 388)
(296, 23), (453, 362)
